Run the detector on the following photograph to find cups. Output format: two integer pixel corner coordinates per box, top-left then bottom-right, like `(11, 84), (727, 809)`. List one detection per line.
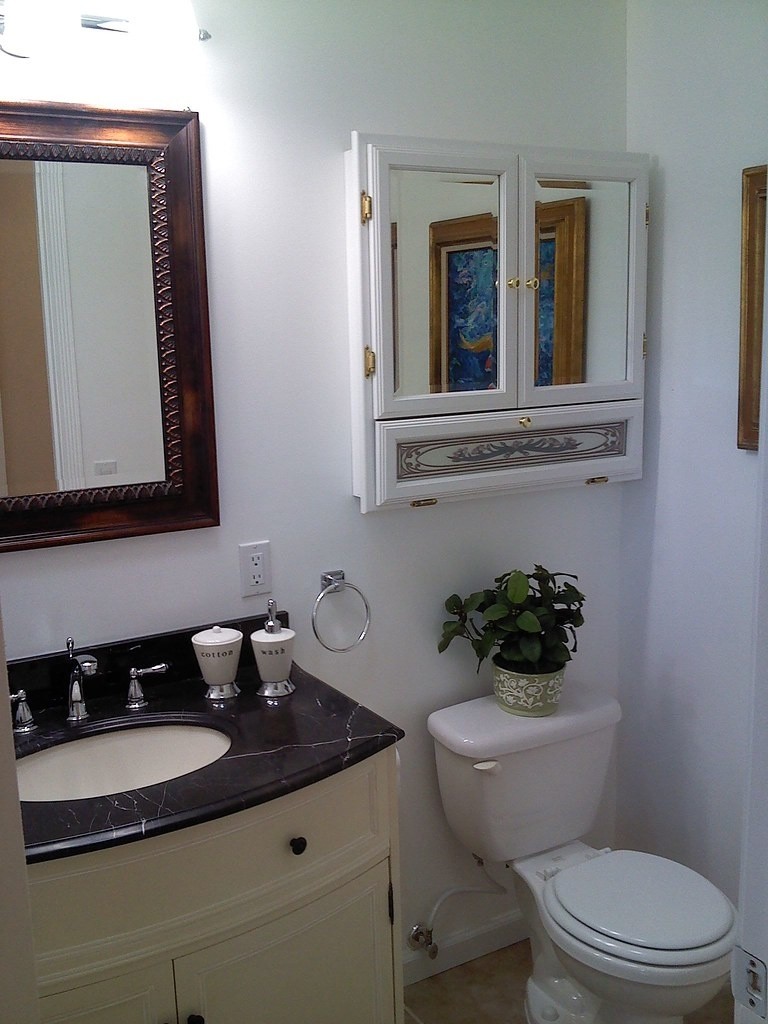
(251, 599), (296, 683)
(190, 625), (243, 685)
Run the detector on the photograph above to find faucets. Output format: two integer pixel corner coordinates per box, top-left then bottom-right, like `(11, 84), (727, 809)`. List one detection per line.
(65, 655), (96, 721)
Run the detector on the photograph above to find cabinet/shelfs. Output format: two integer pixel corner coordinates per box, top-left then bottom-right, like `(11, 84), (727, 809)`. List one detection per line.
(344, 131), (649, 513)
(27, 750), (395, 1024)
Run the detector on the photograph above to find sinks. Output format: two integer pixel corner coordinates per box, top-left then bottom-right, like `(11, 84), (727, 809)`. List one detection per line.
(13, 712), (236, 802)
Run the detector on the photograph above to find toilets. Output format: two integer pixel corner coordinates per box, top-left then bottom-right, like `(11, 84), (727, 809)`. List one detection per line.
(427, 676), (736, 1024)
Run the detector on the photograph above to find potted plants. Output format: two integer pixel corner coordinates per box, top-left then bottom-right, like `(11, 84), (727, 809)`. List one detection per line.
(437, 563), (586, 718)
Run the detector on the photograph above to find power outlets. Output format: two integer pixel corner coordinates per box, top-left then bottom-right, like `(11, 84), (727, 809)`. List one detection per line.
(238, 539), (273, 598)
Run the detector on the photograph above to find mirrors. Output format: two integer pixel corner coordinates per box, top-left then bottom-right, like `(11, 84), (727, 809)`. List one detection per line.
(367, 144), (518, 420)
(0, 101), (220, 553)
(518, 146), (648, 409)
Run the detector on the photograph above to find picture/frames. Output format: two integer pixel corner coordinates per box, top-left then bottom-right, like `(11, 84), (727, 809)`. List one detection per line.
(429, 196), (588, 393)
(737, 165), (768, 450)
